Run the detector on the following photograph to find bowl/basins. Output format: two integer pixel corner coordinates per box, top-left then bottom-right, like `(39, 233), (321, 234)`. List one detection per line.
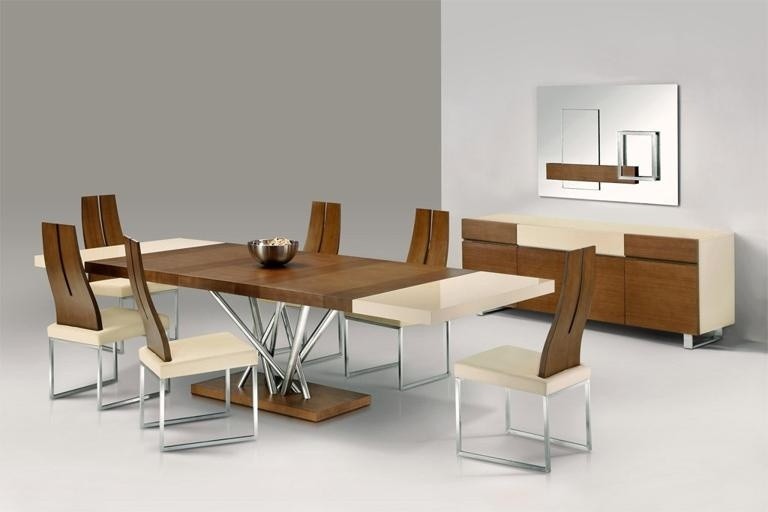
(247, 239), (300, 267)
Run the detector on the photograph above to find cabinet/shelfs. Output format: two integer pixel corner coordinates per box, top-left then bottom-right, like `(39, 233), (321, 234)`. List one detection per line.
(456, 209), (741, 353)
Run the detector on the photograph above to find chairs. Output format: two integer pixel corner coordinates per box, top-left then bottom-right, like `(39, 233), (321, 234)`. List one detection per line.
(245, 199), (347, 373)
(451, 243), (601, 475)
(338, 206), (455, 392)
(75, 189), (181, 358)
(36, 219), (174, 415)
(116, 232), (267, 454)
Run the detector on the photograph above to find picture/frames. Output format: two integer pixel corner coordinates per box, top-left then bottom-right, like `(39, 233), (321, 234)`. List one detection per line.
(617, 130), (661, 181)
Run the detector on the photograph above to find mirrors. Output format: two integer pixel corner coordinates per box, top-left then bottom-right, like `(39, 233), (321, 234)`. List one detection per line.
(562, 109), (601, 190)
(535, 81), (682, 209)
